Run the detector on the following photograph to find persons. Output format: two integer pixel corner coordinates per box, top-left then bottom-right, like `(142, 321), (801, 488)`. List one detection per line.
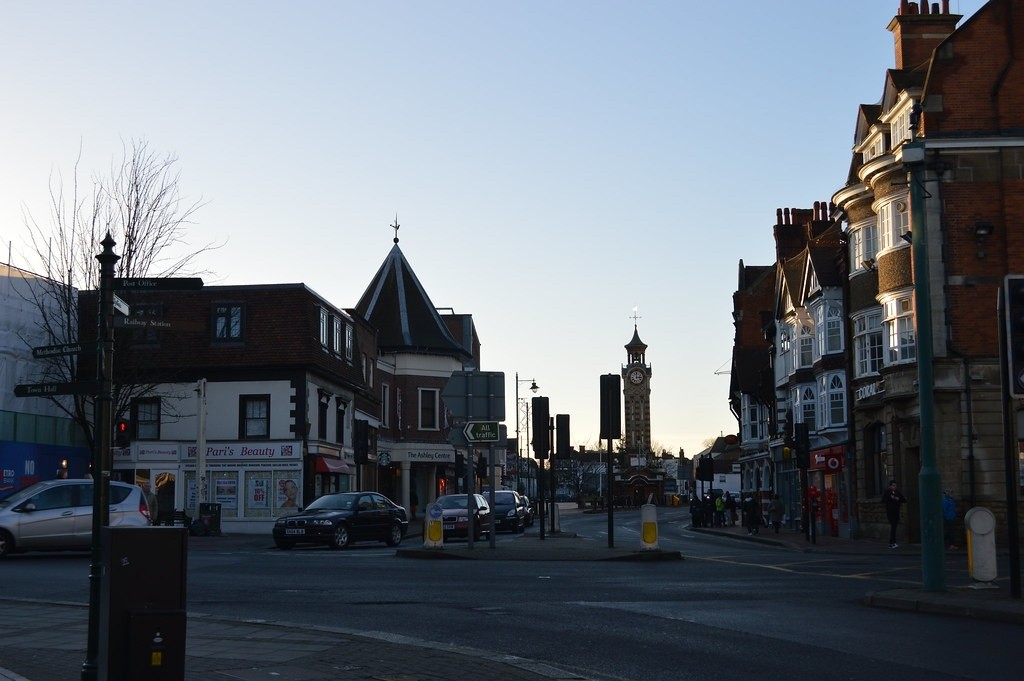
(281, 480), (298, 507)
(689, 491), (736, 528)
(944, 488), (959, 550)
(144, 483), (158, 520)
(743, 495), (760, 536)
(768, 494), (785, 533)
(883, 480), (906, 549)
(410, 488), (418, 519)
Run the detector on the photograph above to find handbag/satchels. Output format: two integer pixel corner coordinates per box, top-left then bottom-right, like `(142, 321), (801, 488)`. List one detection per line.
(770, 505), (775, 510)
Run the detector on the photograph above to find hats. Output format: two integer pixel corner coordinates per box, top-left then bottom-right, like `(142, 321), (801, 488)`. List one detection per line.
(944, 487), (953, 498)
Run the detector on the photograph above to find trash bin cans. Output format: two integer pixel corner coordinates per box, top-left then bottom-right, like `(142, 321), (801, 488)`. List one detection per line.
(96, 525), (187, 680)
(201, 502), (221, 535)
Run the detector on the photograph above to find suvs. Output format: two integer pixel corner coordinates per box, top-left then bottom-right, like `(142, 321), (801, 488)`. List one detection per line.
(482, 490), (525, 534)
(704, 487), (723, 502)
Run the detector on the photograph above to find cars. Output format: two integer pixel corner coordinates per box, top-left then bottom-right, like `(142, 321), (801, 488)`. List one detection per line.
(520, 495), (535, 528)
(0, 478), (151, 561)
(273, 491), (408, 552)
(422, 493), (491, 543)
(722, 491), (741, 506)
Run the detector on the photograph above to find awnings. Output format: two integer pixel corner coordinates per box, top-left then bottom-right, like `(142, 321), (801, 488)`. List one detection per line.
(315, 456), (351, 475)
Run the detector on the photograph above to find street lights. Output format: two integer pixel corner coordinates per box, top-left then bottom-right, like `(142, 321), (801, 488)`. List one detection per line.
(515, 371), (540, 494)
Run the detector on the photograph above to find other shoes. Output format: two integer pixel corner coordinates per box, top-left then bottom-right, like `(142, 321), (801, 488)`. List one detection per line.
(893, 543), (898, 548)
(948, 545), (959, 550)
(888, 544), (895, 548)
(747, 533), (753, 535)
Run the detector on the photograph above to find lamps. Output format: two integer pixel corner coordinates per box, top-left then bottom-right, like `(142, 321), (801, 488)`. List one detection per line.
(900, 231), (912, 244)
(974, 220), (994, 257)
(860, 258), (875, 272)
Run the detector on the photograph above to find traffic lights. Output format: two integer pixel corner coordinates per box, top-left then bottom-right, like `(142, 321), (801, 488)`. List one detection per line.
(116, 419), (130, 447)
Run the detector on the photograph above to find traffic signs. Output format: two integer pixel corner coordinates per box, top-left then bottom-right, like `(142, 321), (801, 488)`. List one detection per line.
(32, 343), (83, 358)
(114, 294), (130, 316)
(13, 382), (73, 398)
(463, 421), (500, 443)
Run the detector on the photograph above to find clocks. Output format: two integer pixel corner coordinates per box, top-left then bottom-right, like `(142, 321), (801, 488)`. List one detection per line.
(628, 368), (646, 385)
